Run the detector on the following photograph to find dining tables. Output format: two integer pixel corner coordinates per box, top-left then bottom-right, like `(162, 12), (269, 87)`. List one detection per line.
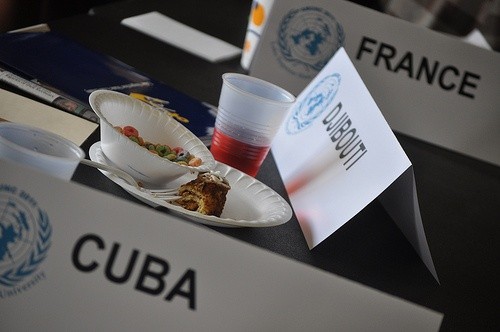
(0, 24), (395, 332)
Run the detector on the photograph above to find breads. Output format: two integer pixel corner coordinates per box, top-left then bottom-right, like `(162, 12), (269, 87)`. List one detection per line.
(172, 171), (230, 217)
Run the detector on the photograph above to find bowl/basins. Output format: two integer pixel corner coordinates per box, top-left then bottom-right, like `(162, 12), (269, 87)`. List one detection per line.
(88, 89), (215, 187)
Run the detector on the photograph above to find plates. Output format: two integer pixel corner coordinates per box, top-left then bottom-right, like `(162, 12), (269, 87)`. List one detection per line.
(88, 139), (292, 230)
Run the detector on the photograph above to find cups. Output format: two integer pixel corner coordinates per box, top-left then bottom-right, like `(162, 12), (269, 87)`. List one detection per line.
(210, 72), (296, 179)
(0, 121), (85, 180)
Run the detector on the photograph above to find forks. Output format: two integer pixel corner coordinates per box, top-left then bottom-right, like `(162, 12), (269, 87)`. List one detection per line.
(80, 159), (182, 203)
